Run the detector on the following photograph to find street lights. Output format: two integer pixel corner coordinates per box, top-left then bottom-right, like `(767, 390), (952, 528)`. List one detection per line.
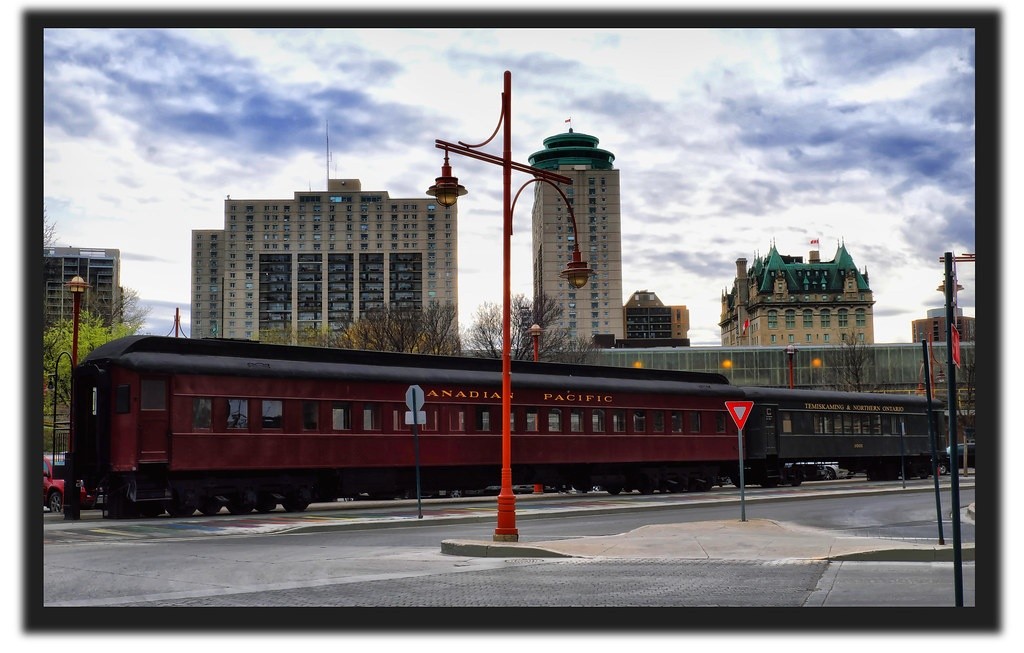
(63, 278), (93, 521)
(425, 71), (595, 544)
(524, 323), (547, 498)
(783, 345), (800, 390)
(937, 251), (965, 608)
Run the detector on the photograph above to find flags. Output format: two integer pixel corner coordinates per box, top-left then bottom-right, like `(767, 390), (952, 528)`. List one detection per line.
(742, 319), (749, 336)
(565, 119), (570, 123)
(811, 239), (819, 244)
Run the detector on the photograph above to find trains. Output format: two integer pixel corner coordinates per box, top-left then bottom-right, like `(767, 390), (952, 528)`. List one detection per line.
(67, 335), (946, 516)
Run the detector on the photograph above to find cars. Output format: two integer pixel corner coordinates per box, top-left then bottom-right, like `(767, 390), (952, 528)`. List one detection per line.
(44, 455), (109, 514)
(931, 443), (976, 475)
(783, 459), (853, 482)
